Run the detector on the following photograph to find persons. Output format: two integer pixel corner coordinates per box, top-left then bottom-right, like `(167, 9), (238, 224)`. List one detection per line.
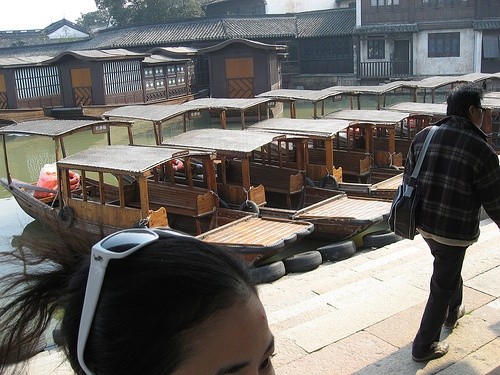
(403, 85), (500, 361)
(0, 221), (277, 375)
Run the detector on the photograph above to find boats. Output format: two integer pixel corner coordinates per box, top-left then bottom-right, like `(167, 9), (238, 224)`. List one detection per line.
(0, 120), (315, 269)
(255, 73), (500, 172)
(102, 104), (394, 243)
(182, 98), (404, 201)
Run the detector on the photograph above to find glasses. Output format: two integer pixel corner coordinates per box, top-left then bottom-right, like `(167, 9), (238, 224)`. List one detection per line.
(478, 106), (487, 112)
(76, 225), (197, 375)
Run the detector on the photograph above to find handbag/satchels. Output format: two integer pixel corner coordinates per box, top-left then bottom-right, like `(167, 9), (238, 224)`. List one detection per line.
(388, 182), (418, 241)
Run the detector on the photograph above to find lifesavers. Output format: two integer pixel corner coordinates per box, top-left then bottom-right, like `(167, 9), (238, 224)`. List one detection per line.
(156, 159), (184, 171)
(69, 171), (80, 191)
(306, 176), (314, 186)
(122, 174), (136, 189)
(57, 207), (75, 229)
(319, 175), (339, 190)
(219, 199), (228, 208)
(132, 224), (148, 228)
(239, 200), (260, 216)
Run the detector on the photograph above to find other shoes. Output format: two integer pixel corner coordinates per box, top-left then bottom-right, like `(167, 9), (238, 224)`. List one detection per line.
(412, 341), (450, 362)
(444, 304), (466, 330)
